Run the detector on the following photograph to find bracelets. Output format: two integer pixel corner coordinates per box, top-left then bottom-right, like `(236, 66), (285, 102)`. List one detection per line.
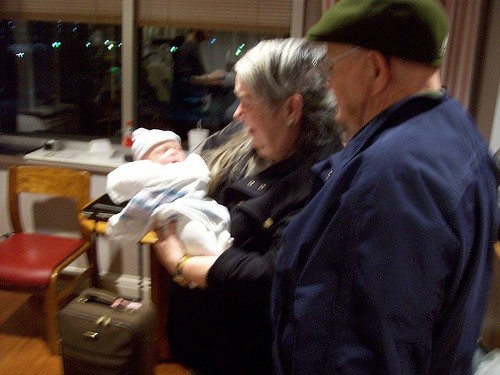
(173, 254), (198, 288)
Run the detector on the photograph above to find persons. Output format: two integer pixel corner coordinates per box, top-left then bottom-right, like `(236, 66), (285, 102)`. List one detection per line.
(271, 0), (500, 375)
(171, 29), (227, 139)
(153, 37), (344, 375)
(106, 127), (234, 288)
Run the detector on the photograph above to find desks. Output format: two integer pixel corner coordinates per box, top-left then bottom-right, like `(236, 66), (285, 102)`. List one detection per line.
(77, 192), (170, 360)
(25, 146), (191, 175)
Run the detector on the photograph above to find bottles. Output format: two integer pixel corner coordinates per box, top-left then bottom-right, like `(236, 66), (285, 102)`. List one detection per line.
(120, 119), (136, 162)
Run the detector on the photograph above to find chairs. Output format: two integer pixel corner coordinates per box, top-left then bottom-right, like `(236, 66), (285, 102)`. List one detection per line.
(0, 163), (100, 357)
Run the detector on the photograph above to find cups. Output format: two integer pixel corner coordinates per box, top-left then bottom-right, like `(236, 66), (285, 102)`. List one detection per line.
(187, 128), (210, 153)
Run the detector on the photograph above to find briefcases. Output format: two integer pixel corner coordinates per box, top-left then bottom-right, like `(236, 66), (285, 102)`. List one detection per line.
(55, 287), (160, 375)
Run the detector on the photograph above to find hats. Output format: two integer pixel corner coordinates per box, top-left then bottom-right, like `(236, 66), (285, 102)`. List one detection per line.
(306, 0), (450, 67)
(131, 128), (181, 161)
